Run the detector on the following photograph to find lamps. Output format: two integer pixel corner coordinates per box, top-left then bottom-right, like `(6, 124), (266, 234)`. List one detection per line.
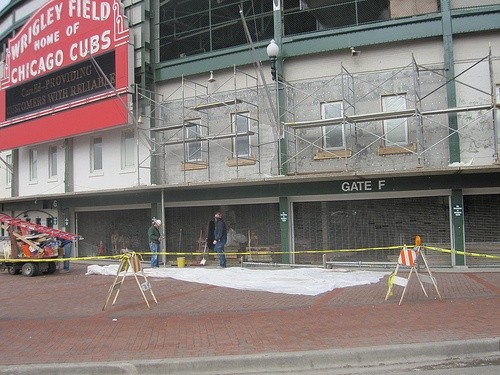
(208, 70), (215, 82)
(138, 116), (143, 125)
(350, 47), (360, 57)
(267, 38), (282, 84)
(65, 218), (69, 225)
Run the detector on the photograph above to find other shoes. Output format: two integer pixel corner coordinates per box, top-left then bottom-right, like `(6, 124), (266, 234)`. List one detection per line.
(217, 266), (224, 268)
(153, 266), (159, 268)
(60, 269), (70, 273)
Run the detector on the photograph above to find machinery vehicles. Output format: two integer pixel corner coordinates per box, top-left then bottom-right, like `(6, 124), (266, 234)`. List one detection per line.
(0, 211), (84, 277)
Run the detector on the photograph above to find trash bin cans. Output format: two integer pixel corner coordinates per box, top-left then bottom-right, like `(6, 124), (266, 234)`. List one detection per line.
(177, 256), (185, 268)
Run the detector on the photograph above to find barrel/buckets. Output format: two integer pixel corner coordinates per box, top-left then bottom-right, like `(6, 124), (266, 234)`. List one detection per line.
(177, 257), (185, 268)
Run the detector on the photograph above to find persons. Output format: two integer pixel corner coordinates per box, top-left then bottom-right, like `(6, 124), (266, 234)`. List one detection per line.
(56, 236), (72, 273)
(213, 212), (227, 268)
(147, 219), (162, 267)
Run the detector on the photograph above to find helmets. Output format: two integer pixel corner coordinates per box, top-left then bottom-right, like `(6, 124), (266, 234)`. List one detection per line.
(213, 213), (222, 219)
(154, 219), (161, 226)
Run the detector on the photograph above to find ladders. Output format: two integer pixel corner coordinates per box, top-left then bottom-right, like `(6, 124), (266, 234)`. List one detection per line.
(0, 213), (83, 241)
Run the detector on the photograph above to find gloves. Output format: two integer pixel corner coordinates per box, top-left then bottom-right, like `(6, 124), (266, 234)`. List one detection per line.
(213, 240), (217, 244)
